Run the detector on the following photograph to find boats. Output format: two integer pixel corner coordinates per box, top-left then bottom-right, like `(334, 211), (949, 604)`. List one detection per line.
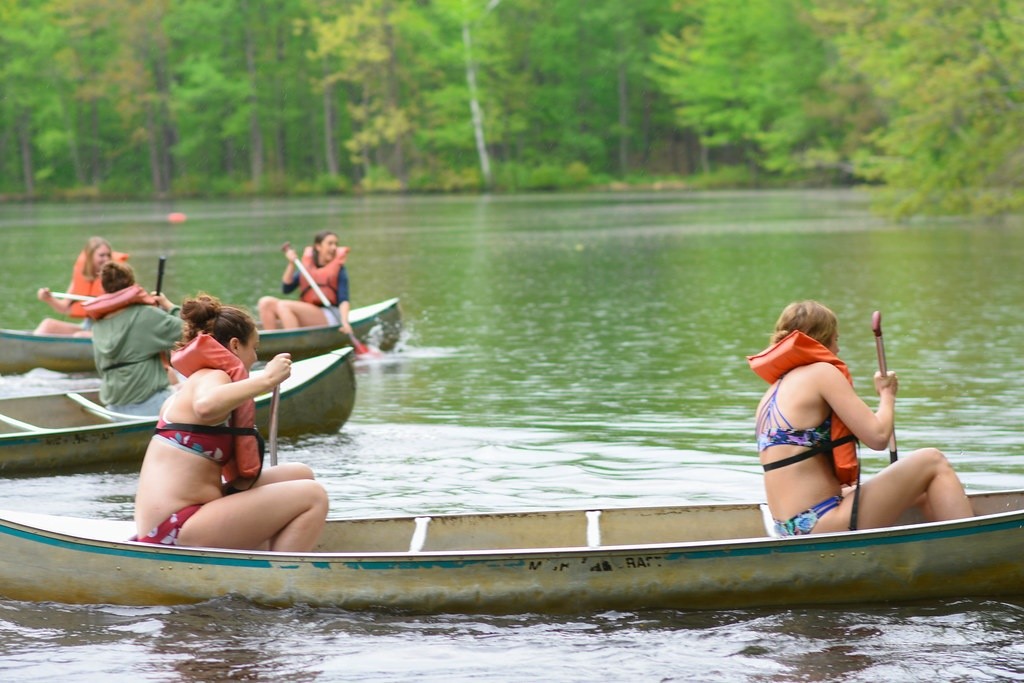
(0, 297), (403, 377)
(1, 347), (357, 474)
(0, 491), (1024, 614)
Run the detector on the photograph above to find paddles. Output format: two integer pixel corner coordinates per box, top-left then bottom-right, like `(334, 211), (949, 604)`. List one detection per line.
(156, 256), (167, 306)
(281, 241), (367, 352)
(50, 292), (97, 301)
(872, 309), (898, 463)
(269, 384), (281, 467)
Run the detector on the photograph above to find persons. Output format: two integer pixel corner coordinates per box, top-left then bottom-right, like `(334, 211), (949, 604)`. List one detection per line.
(745, 300), (974, 537)
(33, 236), (129, 338)
(134, 293), (330, 552)
(258, 231), (355, 336)
(82, 261), (187, 422)
